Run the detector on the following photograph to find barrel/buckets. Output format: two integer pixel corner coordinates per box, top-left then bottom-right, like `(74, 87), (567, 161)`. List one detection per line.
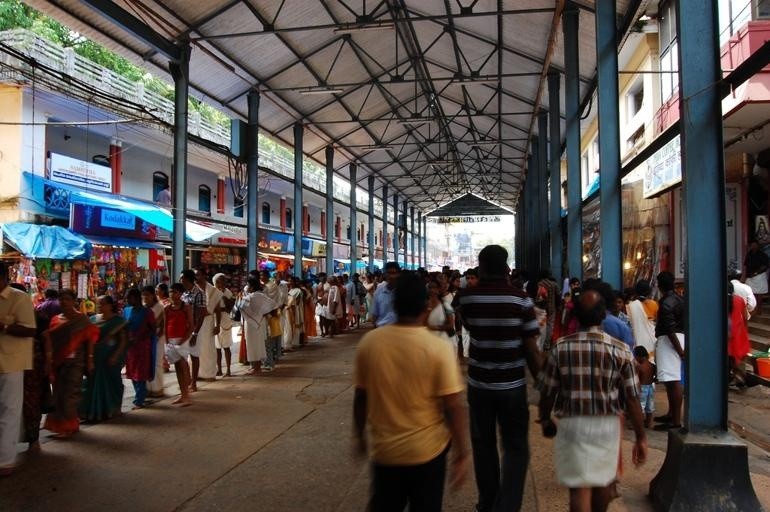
(756, 358), (770, 378)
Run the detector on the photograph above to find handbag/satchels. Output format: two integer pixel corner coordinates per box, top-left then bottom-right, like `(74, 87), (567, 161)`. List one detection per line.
(91, 248), (132, 291)
(445, 318), (461, 337)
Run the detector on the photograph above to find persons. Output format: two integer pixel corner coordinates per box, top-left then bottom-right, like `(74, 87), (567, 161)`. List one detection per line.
(350, 240), (769, 511)
(156, 183), (171, 206)
(153, 267), (290, 406)
(280, 272), (378, 352)
(2, 259), (157, 472)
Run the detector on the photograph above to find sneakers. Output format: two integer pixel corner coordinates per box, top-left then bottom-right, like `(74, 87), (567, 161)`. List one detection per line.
(654, 415), (671, 422)
(653, 420), (681, 431)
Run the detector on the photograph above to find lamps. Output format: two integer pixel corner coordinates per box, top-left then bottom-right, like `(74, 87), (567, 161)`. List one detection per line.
(300, 21), (500, 179)
